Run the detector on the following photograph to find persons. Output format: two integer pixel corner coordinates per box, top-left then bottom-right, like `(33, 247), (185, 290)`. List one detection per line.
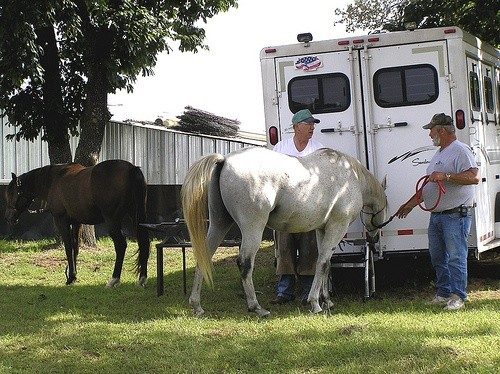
(270, 109), (325, 305)
(395, 112), (480, 311)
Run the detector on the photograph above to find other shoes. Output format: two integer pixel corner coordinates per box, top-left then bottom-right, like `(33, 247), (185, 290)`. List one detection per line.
(271, 296), (295, 304)
(444, 294), (464, 310)
(426, 294), (450, 305)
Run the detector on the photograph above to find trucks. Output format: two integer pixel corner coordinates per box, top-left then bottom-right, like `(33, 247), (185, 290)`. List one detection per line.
(261, 27), (500, 279)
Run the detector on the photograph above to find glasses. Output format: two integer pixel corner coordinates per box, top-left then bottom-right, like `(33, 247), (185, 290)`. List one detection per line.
(302, 122), (316, 126)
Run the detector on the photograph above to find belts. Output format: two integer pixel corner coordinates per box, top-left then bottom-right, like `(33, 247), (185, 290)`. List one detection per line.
(448, 207), (472, 212)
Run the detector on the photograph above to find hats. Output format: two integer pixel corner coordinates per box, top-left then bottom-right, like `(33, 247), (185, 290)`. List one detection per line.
(423, 113), (454, 129)
(292, 109), (320, 125)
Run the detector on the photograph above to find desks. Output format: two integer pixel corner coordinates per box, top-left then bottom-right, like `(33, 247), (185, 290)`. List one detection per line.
(138, 218), (244, 298)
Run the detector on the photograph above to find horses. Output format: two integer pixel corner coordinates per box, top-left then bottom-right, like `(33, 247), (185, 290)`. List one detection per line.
(179, 147), (388, 319)
(6, 159), (149, 290)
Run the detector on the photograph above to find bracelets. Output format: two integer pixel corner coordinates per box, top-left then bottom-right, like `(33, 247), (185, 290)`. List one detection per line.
(445, 172), (451, 183)
